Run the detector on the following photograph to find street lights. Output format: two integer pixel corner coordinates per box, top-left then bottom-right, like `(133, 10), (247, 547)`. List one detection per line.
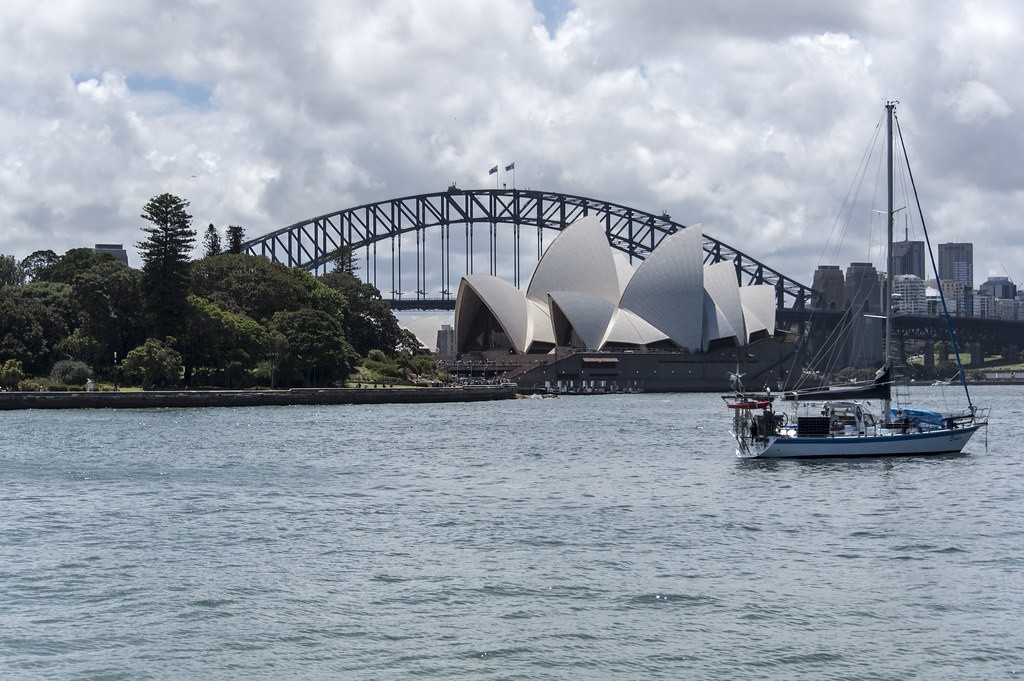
(415, 362), (420, 388)
(484, 358), (488, 379)
(313, 364), (316, 387)
(468, 360), (473, 380)
(455, 360), (462, 378)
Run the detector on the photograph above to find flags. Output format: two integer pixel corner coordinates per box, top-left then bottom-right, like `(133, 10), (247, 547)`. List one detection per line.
(505, 162), (514, 171)
(487, 165), (500, 175)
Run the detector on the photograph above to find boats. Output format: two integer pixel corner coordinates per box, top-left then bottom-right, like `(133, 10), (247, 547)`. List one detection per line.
(931, 380), (951, 386)
(721, 393), (779, 409)
(782, 364), (890, 401)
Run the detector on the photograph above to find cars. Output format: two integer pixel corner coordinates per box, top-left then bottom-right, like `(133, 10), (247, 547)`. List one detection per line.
(470, 380), (476, 385)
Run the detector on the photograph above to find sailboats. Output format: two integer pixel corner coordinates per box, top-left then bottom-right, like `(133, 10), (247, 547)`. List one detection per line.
(720, 100), (992, 461)
